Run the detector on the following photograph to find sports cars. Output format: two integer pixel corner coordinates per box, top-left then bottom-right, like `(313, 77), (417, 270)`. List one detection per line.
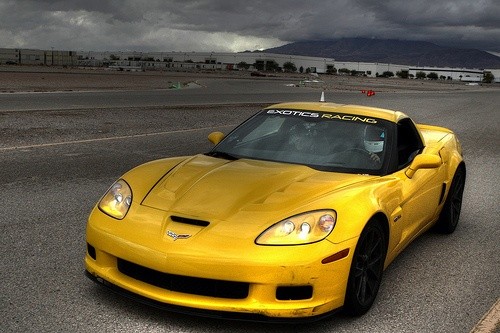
(82, 98), (466, 327)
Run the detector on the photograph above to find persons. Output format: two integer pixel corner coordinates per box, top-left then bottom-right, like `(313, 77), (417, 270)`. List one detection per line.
(287, 123), (316, 154)
(350, 124), (386, 163)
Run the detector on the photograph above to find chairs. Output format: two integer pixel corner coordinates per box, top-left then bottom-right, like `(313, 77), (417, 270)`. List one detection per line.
(279, 124), (385, 163)
